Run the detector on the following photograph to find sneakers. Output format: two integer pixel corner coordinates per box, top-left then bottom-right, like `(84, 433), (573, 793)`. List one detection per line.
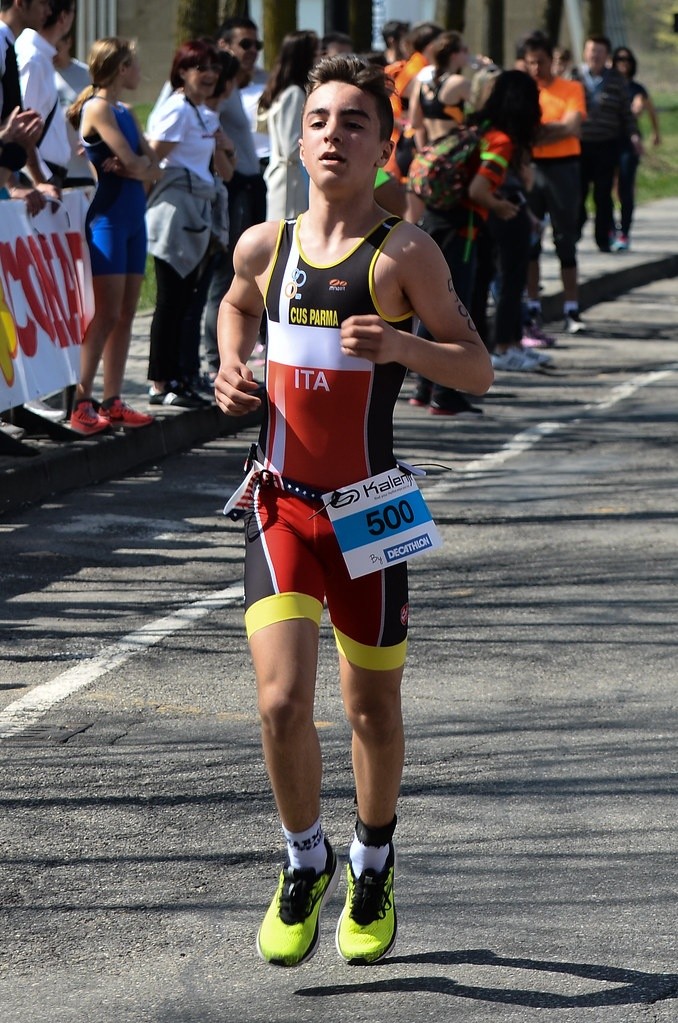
(335, 841), (399, 964)
(562, 311), (585, 333)
(408, 389), (432, 408)
(148, 377), (212, 412)
(429, 395), (483, 416)
(70, 401), (113, 436)
(526, 308), (543, 333)
(98, 400), (155, 429)
(257, 836), (341, 968)
(491, 344), (551, 371)
(521, 327), (553, 348)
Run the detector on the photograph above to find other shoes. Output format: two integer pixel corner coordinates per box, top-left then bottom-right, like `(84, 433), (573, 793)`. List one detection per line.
(612, 233), (627, 251)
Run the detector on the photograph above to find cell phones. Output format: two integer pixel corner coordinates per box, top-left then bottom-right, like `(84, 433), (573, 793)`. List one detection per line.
(507, 192), (526, 207)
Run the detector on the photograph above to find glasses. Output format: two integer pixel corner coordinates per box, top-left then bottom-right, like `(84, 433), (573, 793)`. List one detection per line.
(617, 56), (628, 62)
(228, 38), (264, 50)
(193, 64), (223, 74)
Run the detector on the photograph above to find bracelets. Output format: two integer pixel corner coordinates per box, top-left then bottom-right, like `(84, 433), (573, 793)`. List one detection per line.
(230, 148), (246, 161)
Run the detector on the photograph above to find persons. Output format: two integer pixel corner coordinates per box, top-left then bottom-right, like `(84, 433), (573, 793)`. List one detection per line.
(0, 0), (662, 437)
(215, 57), (495, 967)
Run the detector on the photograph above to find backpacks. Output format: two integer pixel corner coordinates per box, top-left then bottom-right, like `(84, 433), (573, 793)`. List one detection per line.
(405, 119), (498, 214)
